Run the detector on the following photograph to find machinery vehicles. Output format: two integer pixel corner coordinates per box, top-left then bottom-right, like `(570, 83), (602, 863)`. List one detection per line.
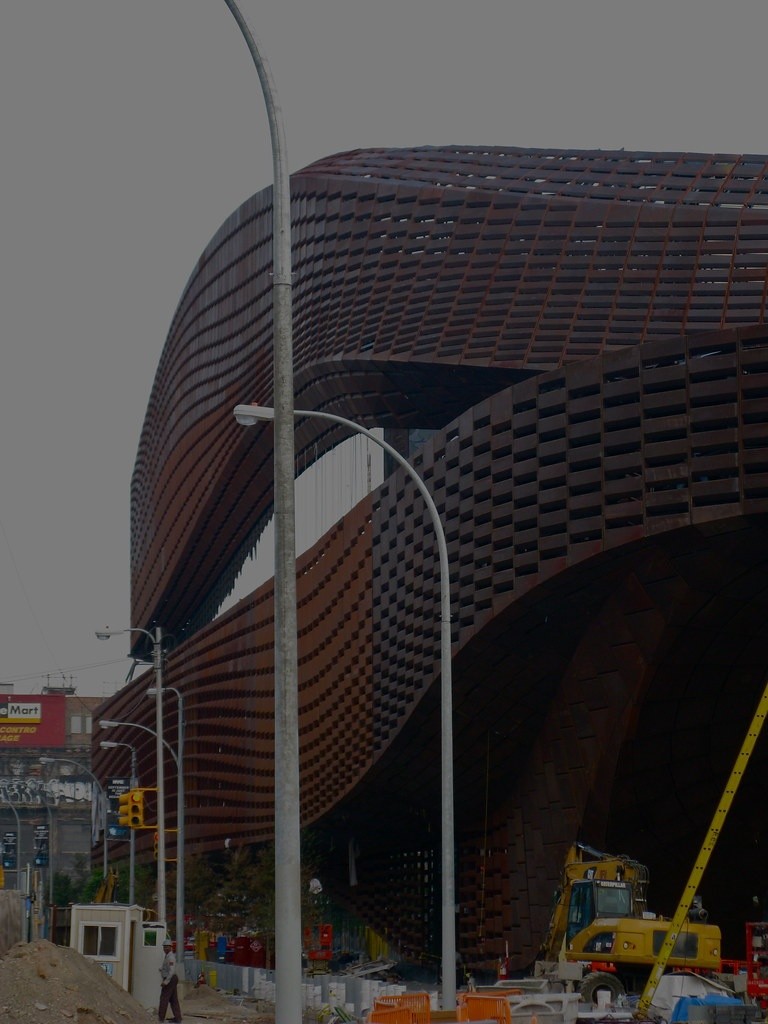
(521, 842), (720, 1000)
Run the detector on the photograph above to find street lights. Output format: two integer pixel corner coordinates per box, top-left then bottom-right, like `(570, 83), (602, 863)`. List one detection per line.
(232, 404), (459, 1013)
(92, 624), (167, 930)
(99, 741), (135, 905)
(0, 781), (53, 944)
(99, 686), (184, 964)
(37, 756), (108, 882)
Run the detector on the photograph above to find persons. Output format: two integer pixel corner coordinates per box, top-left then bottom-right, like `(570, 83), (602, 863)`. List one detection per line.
(158, 939), (182, 1022)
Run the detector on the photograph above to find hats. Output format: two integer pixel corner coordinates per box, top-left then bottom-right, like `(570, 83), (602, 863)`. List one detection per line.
(163, 940), (173, 947)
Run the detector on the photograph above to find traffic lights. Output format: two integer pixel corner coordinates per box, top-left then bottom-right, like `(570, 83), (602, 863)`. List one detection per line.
(118, 792), (129, 825)
(129, 792), (144, 828)
(152, 832), (158, 861)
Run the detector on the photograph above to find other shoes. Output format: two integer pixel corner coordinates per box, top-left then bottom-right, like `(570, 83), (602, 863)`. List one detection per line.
(169, 1018), (180, 1022)
